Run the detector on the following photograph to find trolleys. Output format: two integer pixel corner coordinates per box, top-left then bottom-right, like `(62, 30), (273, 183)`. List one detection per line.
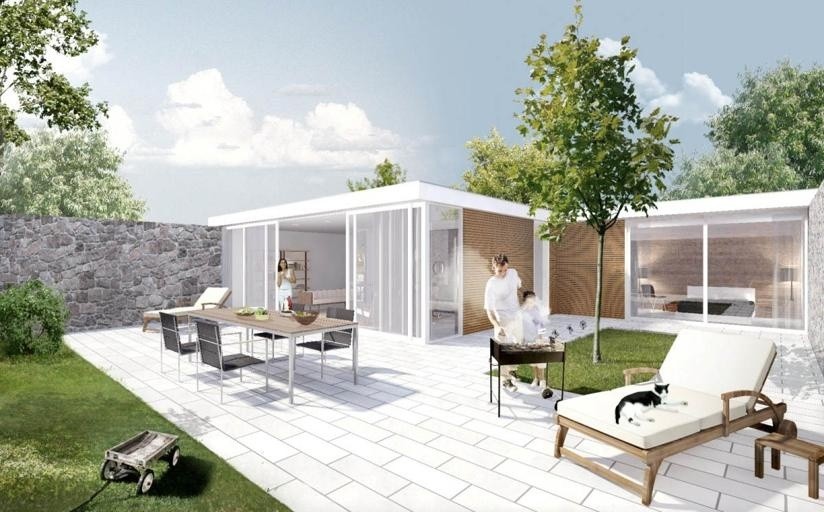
(99, 429), (179, 494)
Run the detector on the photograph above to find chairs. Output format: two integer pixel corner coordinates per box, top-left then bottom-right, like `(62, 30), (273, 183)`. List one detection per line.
(158, 311), (198, 383)
(294, 306), (355, 379)
(193, 317), (269, 405)
(551, 323), (798, 508)
(253, 297), (308, 364)
(141, 285), (232, 349)
(642, 284), (668, 311)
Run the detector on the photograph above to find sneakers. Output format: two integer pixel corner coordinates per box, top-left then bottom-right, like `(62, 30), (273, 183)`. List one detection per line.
(503, 380), (517, 392)
(511, 372), (521, 381)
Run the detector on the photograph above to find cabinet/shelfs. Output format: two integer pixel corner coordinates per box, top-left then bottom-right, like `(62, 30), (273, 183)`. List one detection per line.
(280, 249), (311, 306)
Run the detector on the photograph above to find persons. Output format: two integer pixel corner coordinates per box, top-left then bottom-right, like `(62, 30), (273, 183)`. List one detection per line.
(276, 257), (297, 311)
(484, 253), (526, 394)
(517, 291), (552, 392)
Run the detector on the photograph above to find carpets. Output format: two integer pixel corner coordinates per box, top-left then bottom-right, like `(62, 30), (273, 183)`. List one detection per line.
(663, 300), (732, 315)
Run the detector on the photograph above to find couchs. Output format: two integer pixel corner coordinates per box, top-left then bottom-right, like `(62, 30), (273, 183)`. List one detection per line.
(297, 288), (345, 308)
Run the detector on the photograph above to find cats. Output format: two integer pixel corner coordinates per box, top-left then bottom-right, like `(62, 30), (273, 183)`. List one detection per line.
(615, 384), (689, 426)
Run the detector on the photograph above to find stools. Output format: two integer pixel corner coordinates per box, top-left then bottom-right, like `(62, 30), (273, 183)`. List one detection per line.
(754, 428), (824, 500)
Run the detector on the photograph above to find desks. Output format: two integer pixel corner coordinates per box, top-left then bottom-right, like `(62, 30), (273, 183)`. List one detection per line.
(487, 335), (569, 417)
